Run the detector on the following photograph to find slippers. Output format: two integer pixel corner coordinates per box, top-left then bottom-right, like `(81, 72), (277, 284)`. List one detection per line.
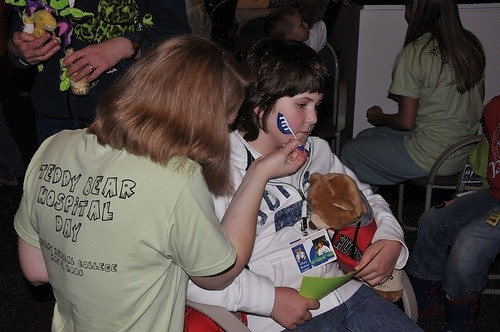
(0, 176), (18, 186)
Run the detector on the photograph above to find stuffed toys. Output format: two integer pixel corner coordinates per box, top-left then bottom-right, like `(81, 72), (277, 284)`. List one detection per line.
(307, 172), (404, 304)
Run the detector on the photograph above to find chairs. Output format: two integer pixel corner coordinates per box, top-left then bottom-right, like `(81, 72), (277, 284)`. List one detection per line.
(312, 43), (341, 157)
(397, 135), (482, 232)
(184, 270), (418, 332)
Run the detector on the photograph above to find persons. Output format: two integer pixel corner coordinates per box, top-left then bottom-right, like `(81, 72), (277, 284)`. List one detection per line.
(0, 0), (349, 186)
(14, 34), (309, 332)
(340, 0), (486, 194)
(405, 94), (500, 332)
(186, 39), (426, 332)
(294, 249), (306, 262)
(315, 241), (330, 256)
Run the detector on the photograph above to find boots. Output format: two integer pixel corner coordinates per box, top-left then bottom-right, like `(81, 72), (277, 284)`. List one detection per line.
(444, 294), (481, 332)
(396, 287), (441, 317)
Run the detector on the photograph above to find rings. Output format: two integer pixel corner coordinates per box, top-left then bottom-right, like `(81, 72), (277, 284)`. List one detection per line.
(88, 63), (95, 70)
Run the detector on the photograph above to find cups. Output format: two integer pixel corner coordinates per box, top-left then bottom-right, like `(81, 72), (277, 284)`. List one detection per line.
(67, 63), (89, 95)
